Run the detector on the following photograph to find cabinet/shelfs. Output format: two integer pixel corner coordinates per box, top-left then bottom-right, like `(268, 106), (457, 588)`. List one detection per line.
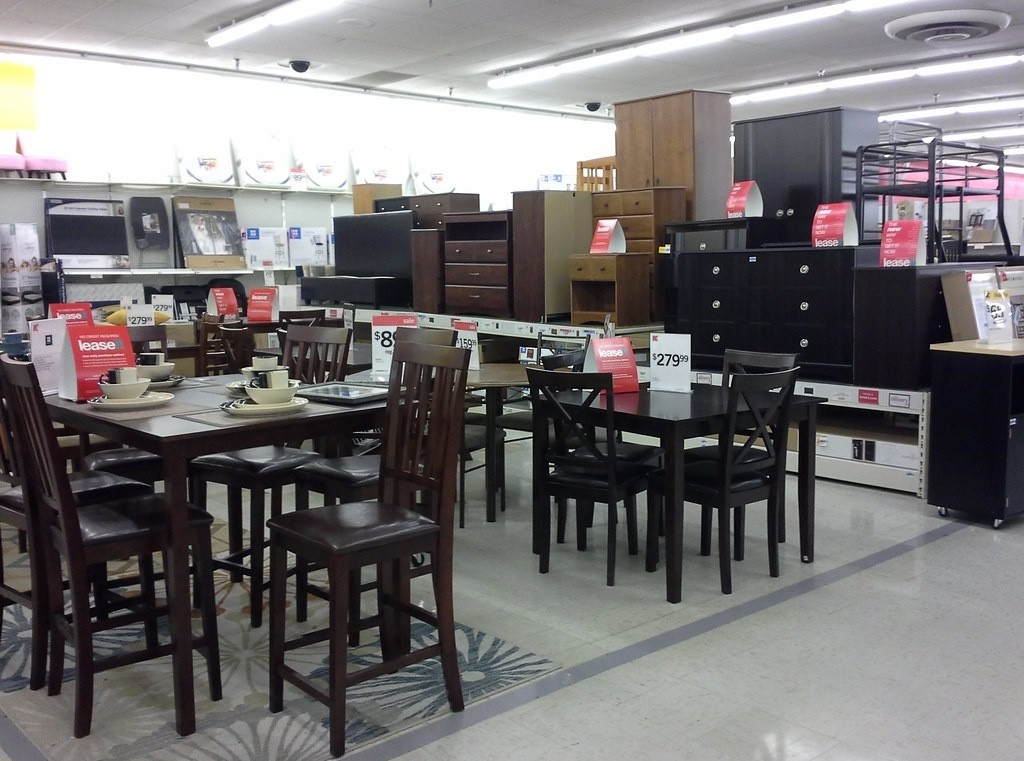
(593, 187), (687, 321)
(664, 218), (880, 385)
(568, 253), (651, 326)
(0, 176), (353, 326)
(442, 210), (511, 321)
(511, 190), (592, 323)
(635, 364), (931, 498)
(352, 184), (402, 214)
(731, 107), (880, 247)
(375, 193), (480, 231)
(298, 306), (665, 416)
(853, 261), (1008, 429)
(856, 139), (1014, 263)
(614, 89), (733, 220)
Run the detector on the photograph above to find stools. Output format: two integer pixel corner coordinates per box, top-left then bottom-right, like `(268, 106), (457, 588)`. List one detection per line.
(0, 156), (67, 180)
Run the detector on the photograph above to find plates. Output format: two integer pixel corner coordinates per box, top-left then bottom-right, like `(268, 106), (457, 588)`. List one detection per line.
(149, 374), (185, 387)
(226, 379), (301, 396)
(219, 396), (309, 417)
(86, 391), (175, 412)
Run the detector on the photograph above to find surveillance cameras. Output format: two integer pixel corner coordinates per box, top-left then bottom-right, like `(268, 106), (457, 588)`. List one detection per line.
(290, 61), (310, 72)
(585, 102), (601, 111)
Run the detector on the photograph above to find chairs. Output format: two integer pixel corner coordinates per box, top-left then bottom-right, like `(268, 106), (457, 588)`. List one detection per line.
(496, 332), (665, 585)
(646, 348), (801, 605)
(0, 309), (506, 758)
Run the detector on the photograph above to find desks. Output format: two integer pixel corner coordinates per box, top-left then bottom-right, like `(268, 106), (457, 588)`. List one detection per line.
(146, 339), (200, 359)
(927, 337), (1023, 528)
(438, 363), (575, 522)
(189, 317), (346, 380)
(255, 343), (373, 374)
(42, 374), (486, 736)
(526, 390), (829, 604)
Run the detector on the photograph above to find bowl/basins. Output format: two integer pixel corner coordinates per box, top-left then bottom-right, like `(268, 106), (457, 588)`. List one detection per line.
(136, 363), (176, 382)
(98, 377), (152, 399)
(244, 384), (299, 405)
(241, 366), (290, 383)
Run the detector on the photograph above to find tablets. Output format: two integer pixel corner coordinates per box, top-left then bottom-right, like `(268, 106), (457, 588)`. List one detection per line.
(297, 382), (407, 403)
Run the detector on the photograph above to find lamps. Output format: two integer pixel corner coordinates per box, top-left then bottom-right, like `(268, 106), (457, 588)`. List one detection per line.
(880, 94), (1024, 157)
(489, 0), (908, 89)
(731, 48), (1024, 104)
(204, 0), (347, 48)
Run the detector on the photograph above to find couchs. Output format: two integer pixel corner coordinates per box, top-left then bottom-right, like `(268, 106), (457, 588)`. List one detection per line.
(302, 210), (418, 311)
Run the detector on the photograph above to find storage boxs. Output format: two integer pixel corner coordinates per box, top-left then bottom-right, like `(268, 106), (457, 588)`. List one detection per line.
(538, 174), (577, 191)
(942, 266), (1024, 342)
(0, 223), (44, 331)
(241, 227), (335, 269)
(179, 137), (458, 194)
(705, 421), (918, 467)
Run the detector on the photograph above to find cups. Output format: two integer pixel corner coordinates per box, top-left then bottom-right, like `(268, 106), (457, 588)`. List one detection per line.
(250, 370), (289, 389)
(99, 366), (138, 384)
(137, 353), (165, 366)
(252, 355), (278, 369)
